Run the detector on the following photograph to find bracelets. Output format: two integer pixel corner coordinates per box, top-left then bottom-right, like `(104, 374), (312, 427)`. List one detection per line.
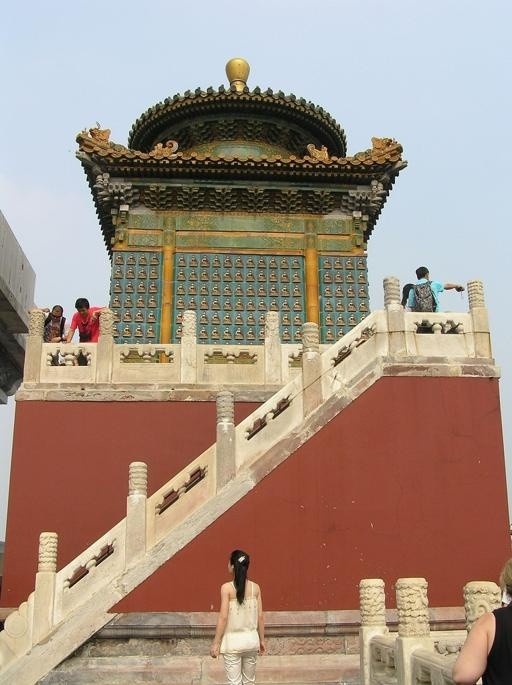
(59, 335), (64, 341)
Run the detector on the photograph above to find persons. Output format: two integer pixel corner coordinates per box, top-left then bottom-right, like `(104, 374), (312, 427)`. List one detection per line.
(65, 298), (110, 366)
(403, 269), (466, 333)
(41, 305), (70, 366)
(209, 551), (266, 685)
(403, 281), (413, 308)
(450, 558), (511, 685)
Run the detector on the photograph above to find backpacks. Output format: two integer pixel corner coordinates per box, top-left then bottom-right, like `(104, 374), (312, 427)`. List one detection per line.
(411, 280), (434, 311)
